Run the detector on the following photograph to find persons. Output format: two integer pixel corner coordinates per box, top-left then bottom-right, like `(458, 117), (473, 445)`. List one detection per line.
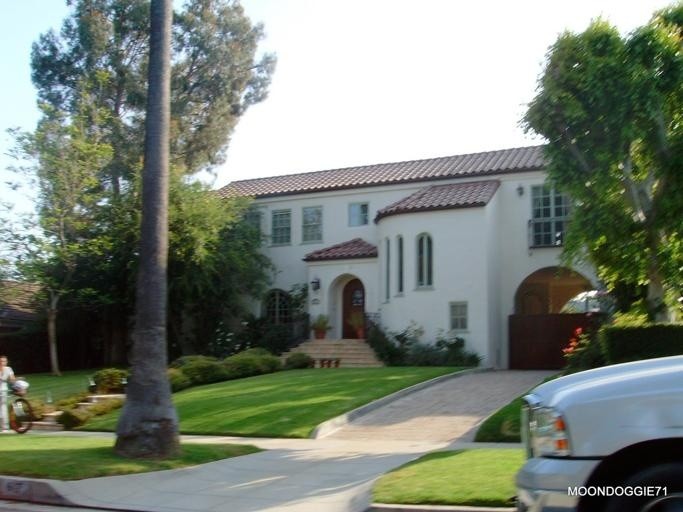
(0, 355), (15, 431)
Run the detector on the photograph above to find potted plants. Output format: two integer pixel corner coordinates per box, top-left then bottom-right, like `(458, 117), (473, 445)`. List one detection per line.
(310, 312), (333, 339)
(346, 310), (371, 338)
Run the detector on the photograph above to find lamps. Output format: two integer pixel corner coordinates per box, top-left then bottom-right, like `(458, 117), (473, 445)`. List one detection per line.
(310, 277), (320, 291)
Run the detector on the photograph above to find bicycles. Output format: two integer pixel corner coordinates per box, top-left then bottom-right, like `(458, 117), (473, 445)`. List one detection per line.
(0, 379), (34, 435)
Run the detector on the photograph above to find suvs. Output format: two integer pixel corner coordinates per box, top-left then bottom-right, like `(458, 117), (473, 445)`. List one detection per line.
(511, 352), (682, 511)
